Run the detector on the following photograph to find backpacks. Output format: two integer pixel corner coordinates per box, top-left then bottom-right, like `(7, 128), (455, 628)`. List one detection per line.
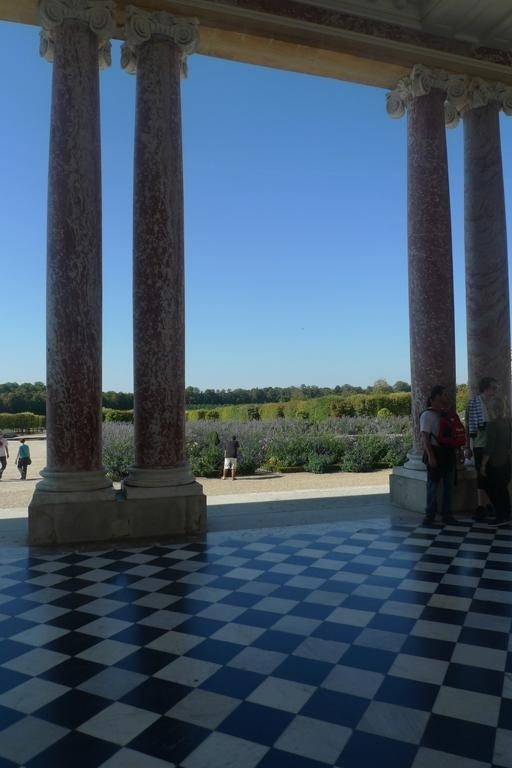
(418, 408), (466, 447)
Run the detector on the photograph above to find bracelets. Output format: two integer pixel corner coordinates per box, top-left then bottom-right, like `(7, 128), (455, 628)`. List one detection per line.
(464, 446), (470, 449)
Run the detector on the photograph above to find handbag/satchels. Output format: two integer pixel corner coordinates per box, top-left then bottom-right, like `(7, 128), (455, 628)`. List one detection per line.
(29, 460), (32, 465)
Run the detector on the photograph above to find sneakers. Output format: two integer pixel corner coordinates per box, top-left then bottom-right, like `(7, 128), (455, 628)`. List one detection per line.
(422, 516), (511, 528)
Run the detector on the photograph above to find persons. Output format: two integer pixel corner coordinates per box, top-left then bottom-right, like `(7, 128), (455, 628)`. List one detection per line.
(481, 395), (512, 525)
(15, 438), (30, 480)
(418, 385), (464, 529)
(220, 435), (240, 480)
(0, 431), (10, 480)
(463, 377), (512, 519)
